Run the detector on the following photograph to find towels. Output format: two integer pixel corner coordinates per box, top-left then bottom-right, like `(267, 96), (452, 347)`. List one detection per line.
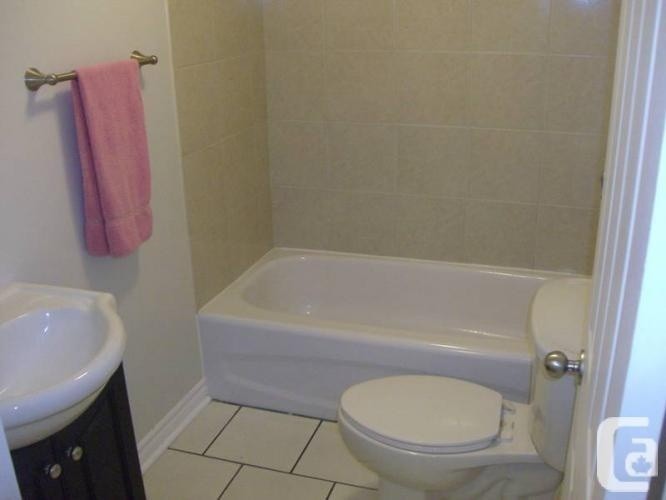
(73, 59), (153, 258)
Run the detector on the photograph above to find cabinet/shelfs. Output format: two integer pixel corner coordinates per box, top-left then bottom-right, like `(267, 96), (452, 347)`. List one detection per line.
(10, 364), (147, 500)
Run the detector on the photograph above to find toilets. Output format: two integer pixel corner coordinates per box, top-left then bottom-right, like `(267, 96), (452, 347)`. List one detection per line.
(337, 278), (588, 500)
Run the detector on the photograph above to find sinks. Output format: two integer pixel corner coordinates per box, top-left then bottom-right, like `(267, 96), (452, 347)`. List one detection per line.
(0, 284), (127, 451)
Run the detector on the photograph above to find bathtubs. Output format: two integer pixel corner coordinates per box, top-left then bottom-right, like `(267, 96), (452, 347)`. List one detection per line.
(195, 246), (592, 424)
